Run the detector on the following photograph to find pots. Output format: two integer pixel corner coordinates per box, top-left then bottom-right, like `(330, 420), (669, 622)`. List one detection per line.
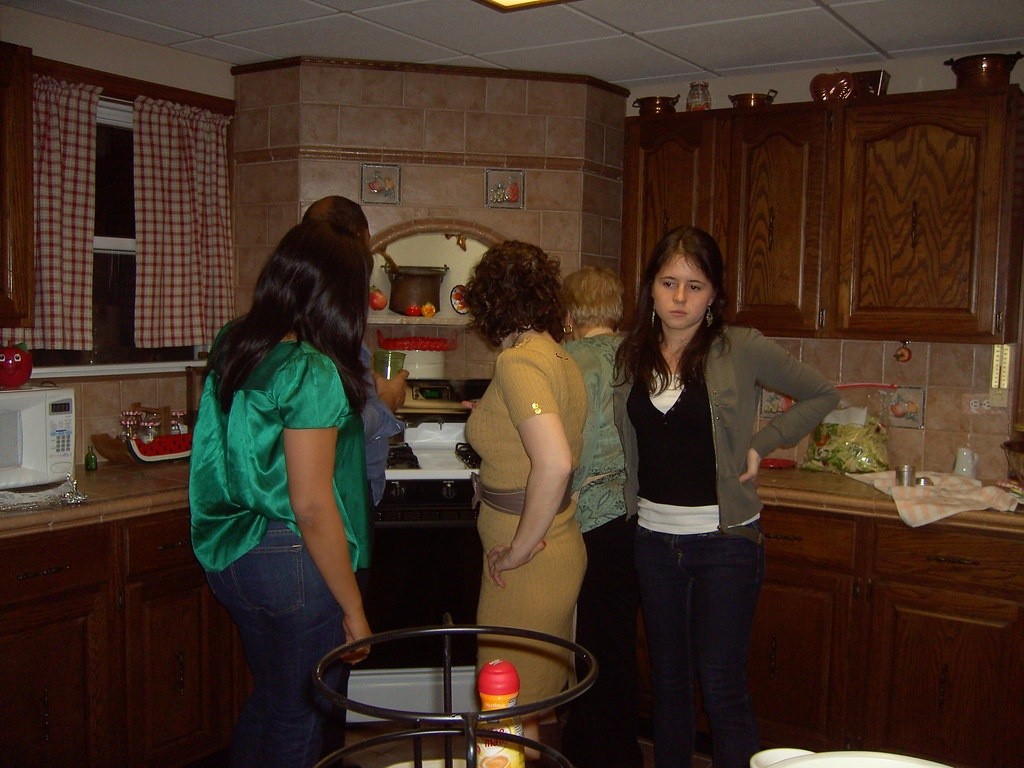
(632, 94), (681, 115)
(943, 51), (1024, 88)
(727, 89), (778, 108)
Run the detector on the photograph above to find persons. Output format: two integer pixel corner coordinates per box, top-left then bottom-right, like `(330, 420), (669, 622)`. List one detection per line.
(608, 222), (842, 768)
(462, 238), (588, 768)
(187, 194), (410, 768)
(551, 264), (646, 768)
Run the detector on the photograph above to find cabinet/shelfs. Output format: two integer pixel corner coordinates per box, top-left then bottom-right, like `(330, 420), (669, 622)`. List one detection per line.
(692, 505), (867, 753)
(0, 522), (120, 768)
(618, 99), (832, 338)
(116, 507), (260, 768)
(851, 519), (1024, 768)
(832, 84), (1024, 344)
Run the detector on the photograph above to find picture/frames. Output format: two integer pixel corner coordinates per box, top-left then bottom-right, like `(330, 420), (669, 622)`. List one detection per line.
(484, 168), (526, 211)
(358, 161), (402, 207)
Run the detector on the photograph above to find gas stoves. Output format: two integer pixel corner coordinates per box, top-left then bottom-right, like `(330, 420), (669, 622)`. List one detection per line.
(383, 379), (493, 508)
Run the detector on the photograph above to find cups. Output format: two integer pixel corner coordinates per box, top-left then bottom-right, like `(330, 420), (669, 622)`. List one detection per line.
(373, 351), (407, 381)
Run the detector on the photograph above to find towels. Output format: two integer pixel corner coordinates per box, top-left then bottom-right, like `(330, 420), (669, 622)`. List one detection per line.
(845, 470), (1019, 528)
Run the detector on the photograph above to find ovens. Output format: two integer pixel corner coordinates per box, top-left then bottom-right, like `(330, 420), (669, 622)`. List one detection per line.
(350, 509), (484, 671)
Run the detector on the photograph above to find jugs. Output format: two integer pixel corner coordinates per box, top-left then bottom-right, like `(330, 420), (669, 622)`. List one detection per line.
(954, 448), (978, 479)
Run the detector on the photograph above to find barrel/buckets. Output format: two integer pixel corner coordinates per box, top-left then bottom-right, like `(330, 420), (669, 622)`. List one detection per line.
(381, 260), (449, 315)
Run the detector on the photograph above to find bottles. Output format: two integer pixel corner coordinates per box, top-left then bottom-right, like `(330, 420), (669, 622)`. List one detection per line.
(122, 410), (143, 420)
(170, 412), (188, 435)
(140, 421), (161, 444)
(85, 445), (97, 470)
(477, 657), (526, 768)
(896, 464), (915, 487)
(118, 421), (140, 441)
(686, 82), (711, 111)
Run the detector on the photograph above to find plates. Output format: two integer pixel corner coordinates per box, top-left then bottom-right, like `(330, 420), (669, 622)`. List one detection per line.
(450, 285), (470, 314)
(759, 458), (798, 468)
(750, 748), (815, 768)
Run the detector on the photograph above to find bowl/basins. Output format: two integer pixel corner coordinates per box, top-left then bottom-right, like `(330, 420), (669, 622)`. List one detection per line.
(764, 751), (952, 768)
(1000, 440), (1024, 482)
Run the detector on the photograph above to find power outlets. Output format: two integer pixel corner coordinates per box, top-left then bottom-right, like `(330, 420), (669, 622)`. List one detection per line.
(961, 393), (1003, 416)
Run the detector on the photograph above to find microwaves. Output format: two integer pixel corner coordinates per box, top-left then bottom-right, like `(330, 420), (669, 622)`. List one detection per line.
(0, 387), (75, 490)
